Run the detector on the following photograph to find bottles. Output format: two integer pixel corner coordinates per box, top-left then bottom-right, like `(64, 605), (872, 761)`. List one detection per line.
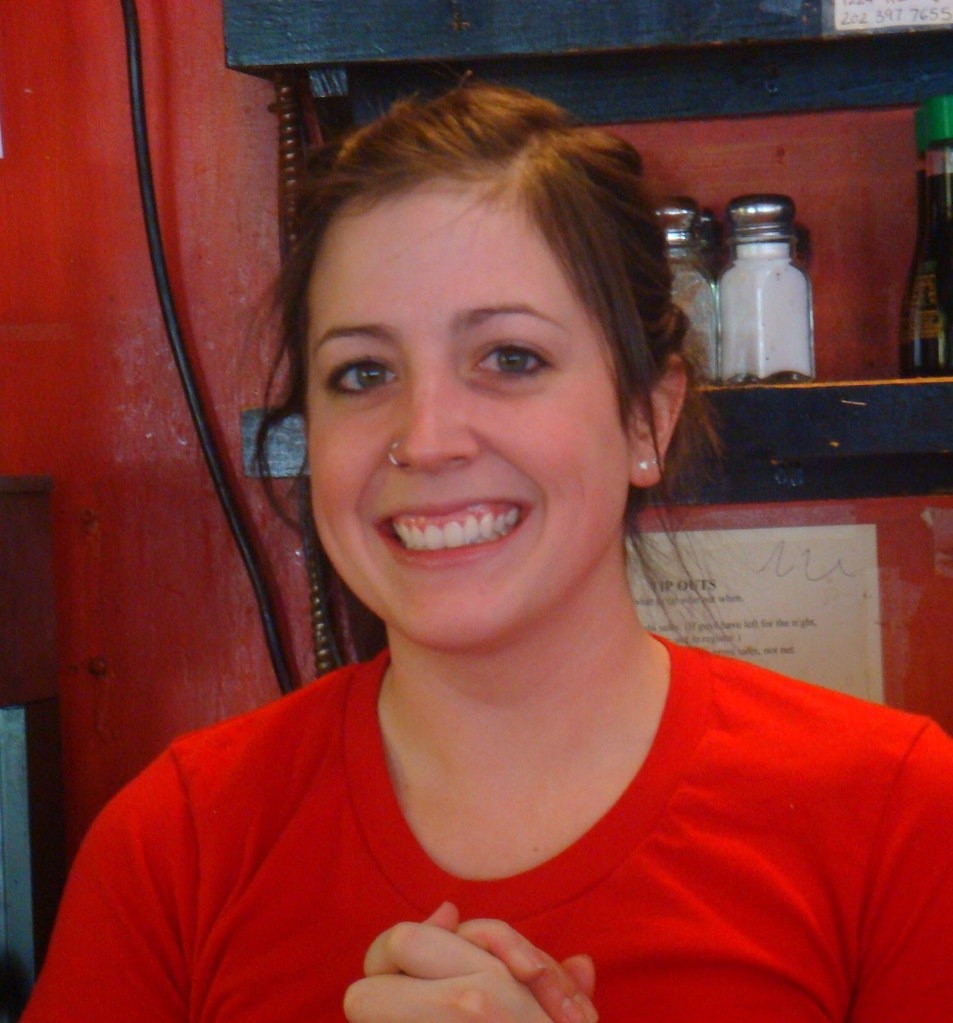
(659, 191), (816, 387)
(907, 92), (952, 378)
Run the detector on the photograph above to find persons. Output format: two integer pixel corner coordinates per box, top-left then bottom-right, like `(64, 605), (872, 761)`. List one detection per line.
(18, 80), (952, 1023)
